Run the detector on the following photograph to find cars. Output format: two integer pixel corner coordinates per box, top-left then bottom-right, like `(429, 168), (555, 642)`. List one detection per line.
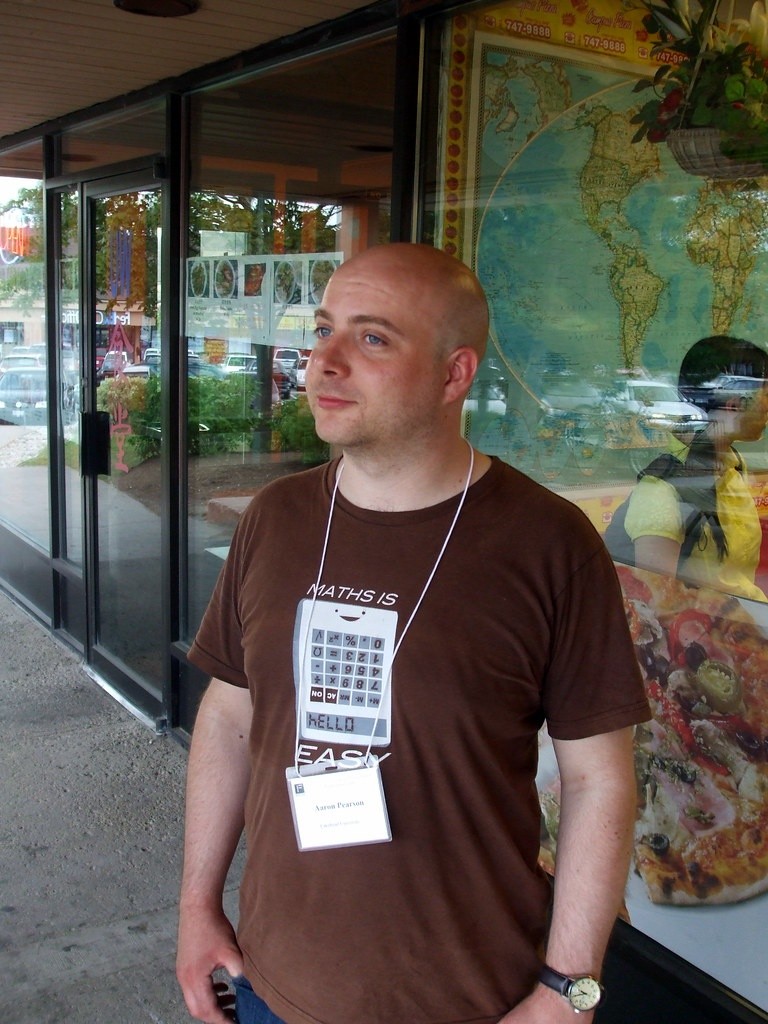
(0, 345), (309, 430)
(465, 363), (768, 433)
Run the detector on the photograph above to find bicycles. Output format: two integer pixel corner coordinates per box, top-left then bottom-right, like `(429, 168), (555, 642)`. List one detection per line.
(557, 383), (681, 481)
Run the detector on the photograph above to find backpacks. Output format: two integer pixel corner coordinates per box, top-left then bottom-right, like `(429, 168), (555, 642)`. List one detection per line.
(602, 455), (707, 567)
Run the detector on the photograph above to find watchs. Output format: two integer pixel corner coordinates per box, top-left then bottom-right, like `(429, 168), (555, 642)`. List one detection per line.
(535, 962), (607, 1014)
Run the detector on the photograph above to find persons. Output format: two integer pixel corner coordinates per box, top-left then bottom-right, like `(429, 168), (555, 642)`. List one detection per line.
(177, 242), (656, 1024)
(624, 336), (768, 607)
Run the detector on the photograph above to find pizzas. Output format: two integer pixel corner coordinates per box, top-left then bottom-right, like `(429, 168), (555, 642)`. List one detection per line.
(531, 563), (768, 923)
